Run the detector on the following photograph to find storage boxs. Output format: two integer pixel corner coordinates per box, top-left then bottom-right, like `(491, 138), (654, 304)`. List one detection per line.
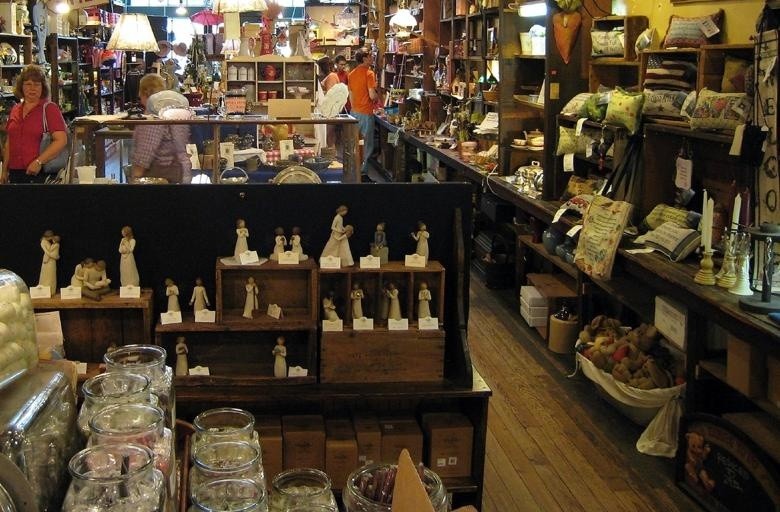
(253, 408), (473, 490)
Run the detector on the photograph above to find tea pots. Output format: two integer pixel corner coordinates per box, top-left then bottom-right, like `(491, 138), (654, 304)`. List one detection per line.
(523, 128), (545, 147)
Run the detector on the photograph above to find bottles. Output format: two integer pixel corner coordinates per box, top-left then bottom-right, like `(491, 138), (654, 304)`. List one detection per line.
(189, 407), (266, 511)
(487, 73), (497, 89)
(227, 65), (254, 80)
(343, 460), (450, 510)
(238, 29), (311, 57)
(529, 216), (578, 268)
(550, 70), (561, 114)
(392, 55), (396, 72)
(476, 74), (484, 100)
(64, 344), (179, 512)
(435, 65), (462, 95)
(269, 468), (339, 511)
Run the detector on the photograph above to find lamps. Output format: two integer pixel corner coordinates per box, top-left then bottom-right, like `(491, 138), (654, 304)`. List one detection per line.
(106, 12), (160, 53)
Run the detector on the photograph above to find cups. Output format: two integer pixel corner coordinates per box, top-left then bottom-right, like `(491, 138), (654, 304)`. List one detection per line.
(513, 139), (527, 146)
(259, 89), (268, 102)
(75, 165), (97, 184)
(386, 64), (394, 71)
(547, 315), (580, 355)
(268, 91), (277, 99)
(528, 94), (539, 103)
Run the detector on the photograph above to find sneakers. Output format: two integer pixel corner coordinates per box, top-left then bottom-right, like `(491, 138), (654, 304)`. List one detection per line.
(361, 174), (377, 184)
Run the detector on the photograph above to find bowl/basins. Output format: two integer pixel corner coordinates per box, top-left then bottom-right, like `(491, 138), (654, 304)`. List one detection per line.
(276, 155), (332, 173)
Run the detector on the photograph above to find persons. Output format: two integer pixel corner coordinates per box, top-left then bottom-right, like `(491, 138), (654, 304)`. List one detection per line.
(175, 336), (189, 376)
(165, 278), (181, 311)
(189, 278), (210, 316)
(123, 73), (192, 184)
(381, 279), (403, 320)
(37, 226), (140, 301)
(0, 65), (67, 184)
(318, 57), (341, 161)
(234, 204), (431, 268)
(335, 55), (352, 162)
(242, 275), (259, 320)
(350, 282), (364, 318)
(322, 290), (340, 320)
(418, 281), (432, 317)
(271, 336), (287, 378)
(348, 48), (382, 183)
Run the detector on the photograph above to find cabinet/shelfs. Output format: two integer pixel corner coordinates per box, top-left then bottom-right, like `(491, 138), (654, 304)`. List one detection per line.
(359, 0), (780, 512)
(68, 111), (360, 185)
(0, 21), (124, 159)
(0, 184), (493, 512)
(221, 59), (315, 105)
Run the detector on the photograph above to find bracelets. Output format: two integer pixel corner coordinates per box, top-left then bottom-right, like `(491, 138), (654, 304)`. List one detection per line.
(36, 158), (42, 165)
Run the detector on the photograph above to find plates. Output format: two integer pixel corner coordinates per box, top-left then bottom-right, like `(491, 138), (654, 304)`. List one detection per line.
(510, 145), (529, 149)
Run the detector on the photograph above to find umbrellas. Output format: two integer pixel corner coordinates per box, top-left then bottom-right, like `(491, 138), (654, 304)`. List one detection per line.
(190, 9), (224, 33)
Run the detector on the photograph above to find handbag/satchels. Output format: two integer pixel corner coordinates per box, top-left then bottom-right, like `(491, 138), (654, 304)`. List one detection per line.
(571, 194), (633, 283)
(37, 124), (83, 176)
